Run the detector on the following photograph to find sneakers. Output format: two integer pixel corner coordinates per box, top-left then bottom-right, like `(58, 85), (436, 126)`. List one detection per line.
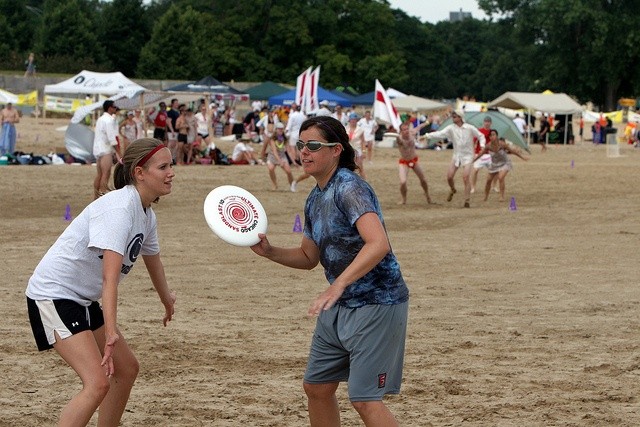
(447, 188), (456, 202)
(290, 180), (297, 192)
(465, 199), (469, 207)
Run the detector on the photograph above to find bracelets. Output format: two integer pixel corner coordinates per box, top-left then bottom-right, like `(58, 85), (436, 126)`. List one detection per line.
(361, 149), (366, 152)
(425, 120), (430, 126)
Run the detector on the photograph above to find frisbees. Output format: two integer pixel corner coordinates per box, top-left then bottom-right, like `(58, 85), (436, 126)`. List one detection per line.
(203, 185), (267, 246)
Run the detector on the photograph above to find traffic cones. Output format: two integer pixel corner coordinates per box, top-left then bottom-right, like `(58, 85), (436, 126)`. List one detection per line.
(64, 204), (72, 221)
(509, 197), (517, 210)
(293, 214), (302, 232)
(570, 160), (574, 168)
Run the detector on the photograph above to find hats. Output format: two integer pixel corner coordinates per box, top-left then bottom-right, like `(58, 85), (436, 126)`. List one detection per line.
(454, 109), (464, 122)
(275, 122), (284, 129)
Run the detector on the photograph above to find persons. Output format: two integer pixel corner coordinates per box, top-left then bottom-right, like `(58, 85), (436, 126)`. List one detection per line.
(256, 112), (281, 165)
(524, 113), (536, 138)
(119, 112), (137, 158)
(537, 116), (549, 144)
(413, 109), (456, 130)
(132, 108), (147, 141)
(348, 105), (360, 128)
(290, 169), (310, 193)
(243, 110), (259, 133)
(93, 100), (119, 198)
(605, 116), (613, 142)
(264, 121), (300, 191)
(288, 102), (297, 118)
(331, 105), (348, 129)
(316, 99), (331, 118)
(110, 107), (122, 165)
(579, 116), (584, 137)
(146, 88), (234, 166)
(618, 96), (640, 149)
(231, 134), (264, 166)
(595, 121), (601, 144)
(0, 102), (19, 161)
(286, 105), (308, 164)
(251, 99), (261, 114)
(345, 112), (367, 182)
(470, 117), (502, 196)
(25, 138), (179, 427)
(547, 114), (555, 131)
(540, 113), (546, 129)
(417, 109), (487, 208)
(358, 111), (379, 160)
(392, 115), (436, 206)
(512, 113), (526, 138)
(472, 130), (529, 203)
(250, 116), (410, 426)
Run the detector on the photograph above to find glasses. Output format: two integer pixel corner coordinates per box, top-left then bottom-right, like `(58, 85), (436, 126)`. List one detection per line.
(296, 140), (346, 154)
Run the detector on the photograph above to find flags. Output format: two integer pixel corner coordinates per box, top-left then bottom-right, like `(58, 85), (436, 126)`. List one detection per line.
(371, 78), (403, 134)
(309, 63), (321, 115)
(295, 66), (312, 116)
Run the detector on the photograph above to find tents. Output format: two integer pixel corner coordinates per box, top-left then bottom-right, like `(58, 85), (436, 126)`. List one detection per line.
(436, 110), (529, 157)
